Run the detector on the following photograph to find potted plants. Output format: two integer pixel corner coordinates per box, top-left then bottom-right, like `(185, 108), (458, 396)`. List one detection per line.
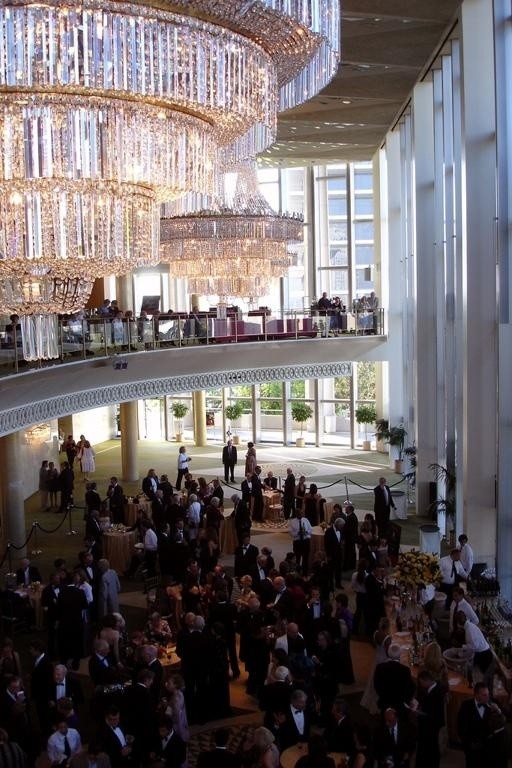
(290, 401), (313, 447)
(224, 402), (244, 444)
(169, 401), (190, 442)
(356, 404), (377, 451)
(386, 426), (406, 473)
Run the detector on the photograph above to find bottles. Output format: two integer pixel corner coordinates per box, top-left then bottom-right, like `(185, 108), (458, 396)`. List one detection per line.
(472, 597), (511, 672)
(391, 579), (434, 667)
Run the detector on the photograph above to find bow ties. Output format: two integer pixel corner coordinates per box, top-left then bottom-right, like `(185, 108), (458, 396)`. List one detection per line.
(56, 682), (65, 687)
(478, 703), (487, 708)
(242, 547), (248, 551)
(295, 710), (302, 714)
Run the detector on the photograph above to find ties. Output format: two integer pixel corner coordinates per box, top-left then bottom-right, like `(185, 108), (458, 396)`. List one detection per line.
(450, 560), (458, 581)
(452, 603), (458, 630)
(298, 519), (304, 540)
(64, 737), (71, 759)
(390, 728), (395, 746)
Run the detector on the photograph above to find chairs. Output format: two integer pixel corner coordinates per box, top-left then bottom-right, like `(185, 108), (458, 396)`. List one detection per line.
(0, 304), (377, 373)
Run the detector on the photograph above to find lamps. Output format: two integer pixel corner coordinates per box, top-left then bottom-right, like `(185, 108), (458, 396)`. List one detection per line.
(1, 0), (343, 361)
(156, 158), (311, 314)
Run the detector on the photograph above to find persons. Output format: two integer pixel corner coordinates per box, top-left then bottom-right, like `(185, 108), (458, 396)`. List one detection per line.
(358, 292), (377, 335)
(0, 435), (510, 768)
(137, 306), (214, 347)
(59, 298), (136, 356)
(8, 314), (27, 368)
(318, 292), (344, 338)
